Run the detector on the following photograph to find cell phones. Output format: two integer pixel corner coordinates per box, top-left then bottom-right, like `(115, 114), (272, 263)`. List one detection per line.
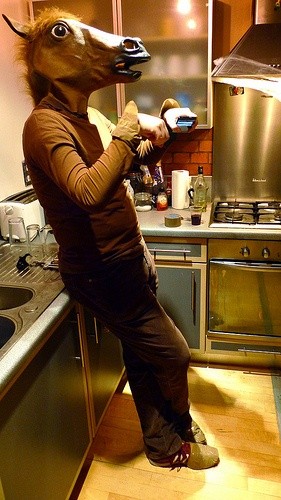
(175, 116), (197, 131)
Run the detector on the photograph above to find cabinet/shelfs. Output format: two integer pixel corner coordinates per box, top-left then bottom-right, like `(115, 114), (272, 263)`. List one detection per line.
(151, 262), (207, 353)
(29, 2), (214, 132)
(0, 295), (127, 500)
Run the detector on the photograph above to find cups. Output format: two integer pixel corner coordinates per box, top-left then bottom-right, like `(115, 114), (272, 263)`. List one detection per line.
(26, 225), (45, 258)
(9, 218), (29, 257)
(190, 206), (202, 224)
(42, 224), (60, 256)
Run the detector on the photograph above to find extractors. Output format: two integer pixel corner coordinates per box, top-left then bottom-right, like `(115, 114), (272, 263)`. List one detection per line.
(211, 0), (281, 79)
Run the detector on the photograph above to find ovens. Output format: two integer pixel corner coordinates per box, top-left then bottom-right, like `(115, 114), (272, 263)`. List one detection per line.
(208, 261), (281, 357)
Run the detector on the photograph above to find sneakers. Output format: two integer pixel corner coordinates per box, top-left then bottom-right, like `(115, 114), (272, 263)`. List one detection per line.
(148, 441), (220, 470)
(181, 420), (207, 444)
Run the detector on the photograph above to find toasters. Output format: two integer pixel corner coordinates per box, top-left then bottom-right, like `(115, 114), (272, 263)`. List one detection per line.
(0, 189), (45, 243)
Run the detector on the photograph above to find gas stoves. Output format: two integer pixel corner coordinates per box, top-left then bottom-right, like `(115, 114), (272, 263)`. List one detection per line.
(209, 199), (281, 262)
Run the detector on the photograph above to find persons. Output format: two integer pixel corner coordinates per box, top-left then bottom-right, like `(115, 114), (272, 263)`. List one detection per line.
(2, 7), (219, 469)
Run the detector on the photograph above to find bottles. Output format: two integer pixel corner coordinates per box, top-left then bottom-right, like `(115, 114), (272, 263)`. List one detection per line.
(135, 193), (151, 211)
(193, 166), (207, 212)
(157, 187), (167, 211)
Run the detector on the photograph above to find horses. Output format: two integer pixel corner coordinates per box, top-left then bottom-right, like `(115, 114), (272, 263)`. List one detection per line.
(2, 9), (150, 116)
